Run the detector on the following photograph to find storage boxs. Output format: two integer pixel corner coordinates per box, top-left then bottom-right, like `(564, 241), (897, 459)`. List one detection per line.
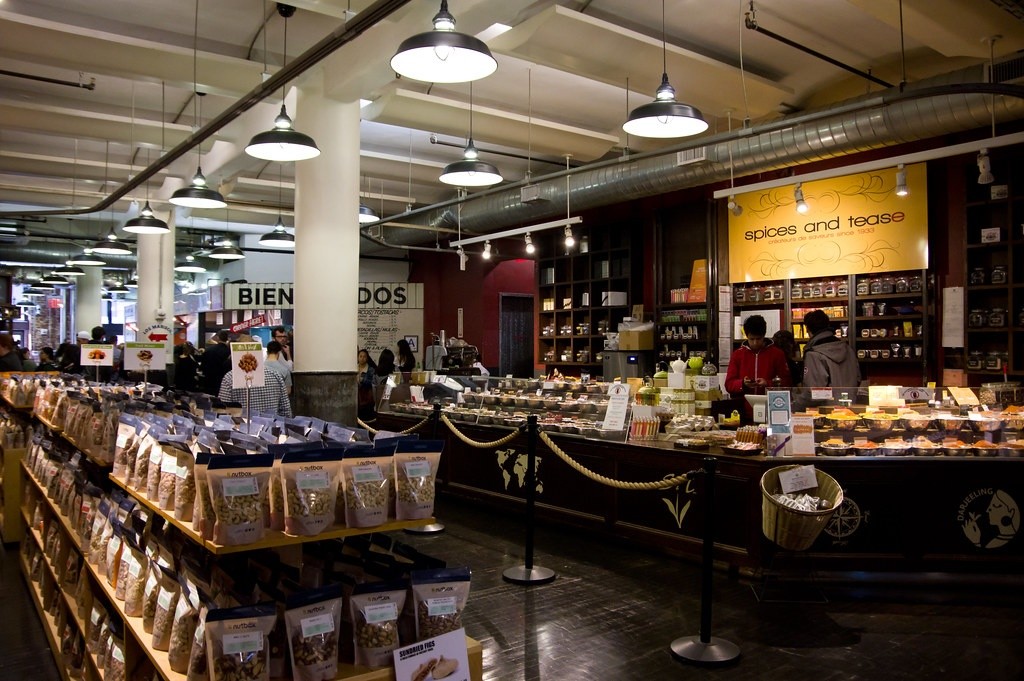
(619, 329), (654, 351)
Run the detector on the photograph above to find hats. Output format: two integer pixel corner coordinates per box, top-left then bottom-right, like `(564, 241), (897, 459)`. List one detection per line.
(76, 331), (93, 341)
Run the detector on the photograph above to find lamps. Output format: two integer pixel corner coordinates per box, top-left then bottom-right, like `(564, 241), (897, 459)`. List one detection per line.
(794, 182), (808, 212)
(389, 0), (498, 84)
(525, 234), (536, 252)
(258, 161), (295, 247)
(208, 207), (246, 259)
(16, 186), (133, 307)
(172, 214), (207, 273)
(359, 205), (380, 224)
(728, 194), (743, 216)
(245, 3), (320, 161)
(895, 164), (907, 197)
(168, 96), (227, 210)
(623, 0), (709, 139)
(976, 147), (994, 184)
(565, 224), (575, 247)
(122, 149), (171, 234)
(438, 79), (502, 187)
(482, 243), (492, 260)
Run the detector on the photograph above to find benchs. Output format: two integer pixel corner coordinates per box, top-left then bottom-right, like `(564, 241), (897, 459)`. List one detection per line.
(767, 386), (1024, 609)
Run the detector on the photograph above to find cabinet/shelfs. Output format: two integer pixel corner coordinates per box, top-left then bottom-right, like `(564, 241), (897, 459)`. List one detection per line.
(0, 370), (83, 550)
(607, 428), (766, 593)
(852, 269), (936, 385)
(20, 408), (483, 681)
(657, 303), (718, 372)
(377, 371), (638, 552)
(731, 274), (788, 356)
(789, 274), (852, 364)
(964, 199), (1024, 386)
(537, 222), (644, 379)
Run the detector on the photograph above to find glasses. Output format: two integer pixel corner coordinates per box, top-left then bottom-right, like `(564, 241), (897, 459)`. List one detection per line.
(274, 335), (289, 339)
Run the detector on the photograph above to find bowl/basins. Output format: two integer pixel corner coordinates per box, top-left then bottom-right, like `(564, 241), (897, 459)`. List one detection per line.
(797, 407), (1024, 456)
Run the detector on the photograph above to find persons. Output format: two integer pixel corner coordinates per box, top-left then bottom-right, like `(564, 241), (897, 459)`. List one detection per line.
(393, 339), (415, 382)
(218, 335), (293, 420)
(772, 331), (805, 388)
(470, 352), (490, 392)
(724, 315), (792, 423)
(1, 311), (294, 397)
(792, 310), (862, 407)
(357, 349), (378, 439)
(371, 348), (404, 411)
